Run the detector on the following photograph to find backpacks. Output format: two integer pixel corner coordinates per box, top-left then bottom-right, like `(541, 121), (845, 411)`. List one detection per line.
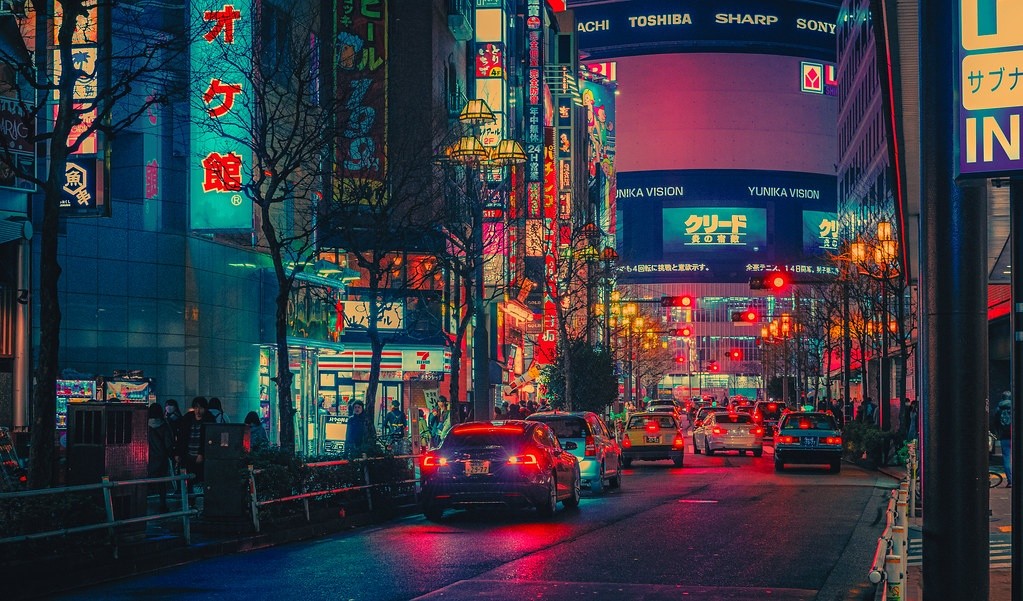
(391, 411), (404, 434)
(997, 407), (1012, 426)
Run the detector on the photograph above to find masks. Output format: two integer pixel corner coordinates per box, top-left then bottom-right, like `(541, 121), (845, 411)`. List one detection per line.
(165, 406), (177, 413)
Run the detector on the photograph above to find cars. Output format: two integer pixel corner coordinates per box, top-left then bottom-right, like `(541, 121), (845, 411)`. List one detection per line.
(692, 412), (764, 458)
(645, 395), (787, 458)
(420, 418), (583, 522)
(524, 409), (622, 497)
(618, 411), (686, 470)
(771, 410), (844, 474)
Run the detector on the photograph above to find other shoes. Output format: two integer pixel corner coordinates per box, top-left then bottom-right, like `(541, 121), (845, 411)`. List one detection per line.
(1006, 484), (1012, 488)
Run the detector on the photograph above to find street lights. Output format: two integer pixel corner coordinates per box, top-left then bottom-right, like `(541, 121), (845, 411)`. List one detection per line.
(760, 313), (804, 406)
(445, 99), (530, 419)
(594, 291), (668, 411)
(559, 223), (618, 346)
(851, 219), (901, 432)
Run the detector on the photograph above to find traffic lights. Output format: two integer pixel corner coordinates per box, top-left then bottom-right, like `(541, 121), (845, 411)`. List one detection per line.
(660, 295), (693, 307)
(761, 275), (788, 289)
(672, 357), (684, 362)
(706, 365), (719, 371)
(731, 310), (758, 323)
(725, 351), (743, 358)
(669, 328), (690, 336)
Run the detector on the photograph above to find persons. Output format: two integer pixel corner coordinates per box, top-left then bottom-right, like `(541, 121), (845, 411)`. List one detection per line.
(904, 398), (919, 442)
(418, 409), (430, 443)
(818, 394), (878, 425)
(427, 395), (451, 449)
(317, 396), (330, 421)
(383, 400), (407, 437)
(345, 399), (377, 450)
(994, 391), (1012, 487)
(494, 398), (552, 420)
(244, 411), (270, 450)
(145, 395), (230, 515)
